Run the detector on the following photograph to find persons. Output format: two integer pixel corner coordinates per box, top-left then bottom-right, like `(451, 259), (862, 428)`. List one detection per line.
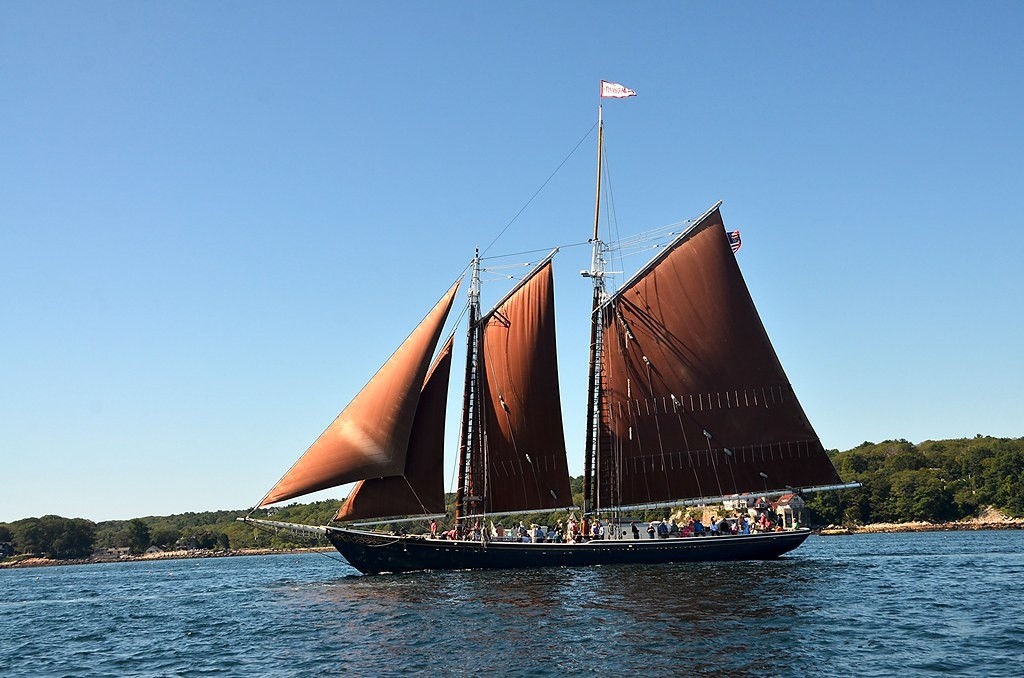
(430, 518), (437, 539)
(441, 521), (492, 548)
(730, 512), (783, 535)
(658, 520), (695, 538)
(605, 517), (615, 540)
(496, 524), (504, 536)
(511, 523), (518, 536)
(720, 518), (731, 532)
(791, 518), (799, 529)
(537, 528), (544, 542)
(631, 521), (640, 539)
(710, 521), (721, 536)
(519, 518), (604, 544)
(694, 520), (706, 537)
(645, 524), (655, 538)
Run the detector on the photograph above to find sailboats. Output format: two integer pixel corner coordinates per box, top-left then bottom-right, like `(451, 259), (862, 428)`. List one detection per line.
(235, 78), (866, 569)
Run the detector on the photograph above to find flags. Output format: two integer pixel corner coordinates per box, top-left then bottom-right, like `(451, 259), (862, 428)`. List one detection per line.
(727, 231), (741, 253)
(601, 80), (637, 99)
